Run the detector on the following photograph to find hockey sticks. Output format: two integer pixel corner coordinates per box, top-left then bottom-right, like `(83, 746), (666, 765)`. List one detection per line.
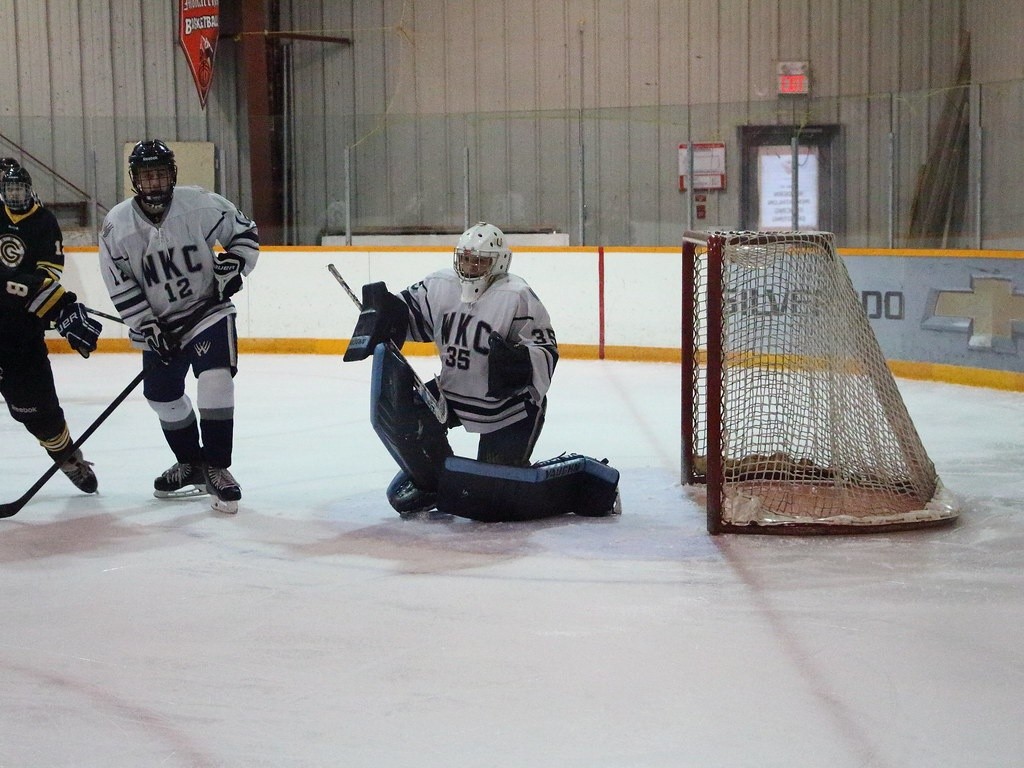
(0, 274), (243, 519)
(327, 263), (450, 425)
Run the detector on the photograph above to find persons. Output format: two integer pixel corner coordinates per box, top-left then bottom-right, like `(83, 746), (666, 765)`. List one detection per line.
(99, 140), (261, 512)
(343, 219), (623, 520)
(0, 158), (98, 495)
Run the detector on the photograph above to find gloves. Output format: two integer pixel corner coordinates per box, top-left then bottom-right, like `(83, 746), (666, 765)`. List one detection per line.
(54, 303), (103, 359)
(140, 317), (181, 369)
(214, 253), (245, 301)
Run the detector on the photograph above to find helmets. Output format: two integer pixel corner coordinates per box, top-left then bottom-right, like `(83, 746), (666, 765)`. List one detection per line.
(0, 167), (34, 214)
(128, 139), (178, 209)
(453, 221), (511, 303)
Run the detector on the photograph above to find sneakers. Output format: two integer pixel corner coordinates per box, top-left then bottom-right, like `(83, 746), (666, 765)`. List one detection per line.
(43, 437), (99, 495)
(153, 462), (208, 498)
(202, 462), (242, 514)
